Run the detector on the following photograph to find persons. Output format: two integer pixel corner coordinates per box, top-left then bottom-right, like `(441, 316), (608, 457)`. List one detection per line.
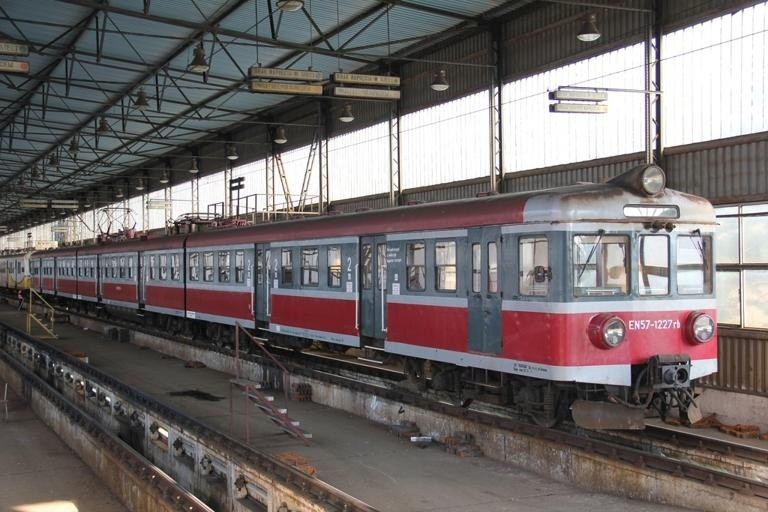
(17, 284), (26, 311)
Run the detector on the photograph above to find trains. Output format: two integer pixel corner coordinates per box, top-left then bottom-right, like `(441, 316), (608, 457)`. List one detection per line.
(0, 163), (720, 430)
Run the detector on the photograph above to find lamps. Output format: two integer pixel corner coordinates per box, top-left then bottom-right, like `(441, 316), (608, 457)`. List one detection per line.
(576, 11), (602, 42)
(275, 1), (305, 12)
(430, 66), (449, 91)
(3, 79), (242, 193)
(339, 103), (355, 124)
(273, 128), (288, 145)
(188, 42), (210, 74)
